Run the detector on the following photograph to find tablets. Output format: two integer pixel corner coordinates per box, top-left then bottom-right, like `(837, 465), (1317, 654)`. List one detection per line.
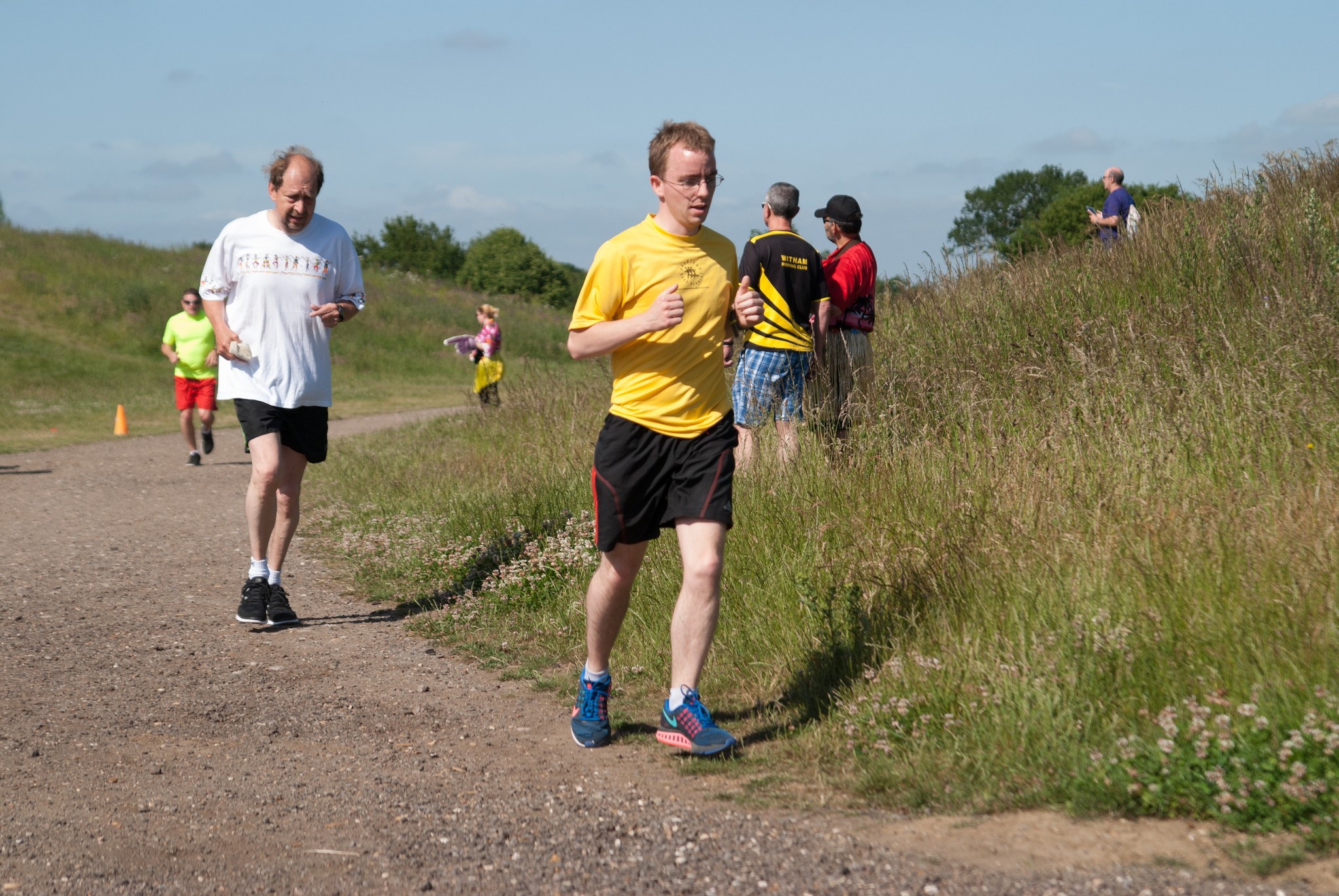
(1086, 205), (1097, 215)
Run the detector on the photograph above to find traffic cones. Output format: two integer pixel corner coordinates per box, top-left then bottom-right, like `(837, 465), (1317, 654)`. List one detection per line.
(112, 404), (128, 437)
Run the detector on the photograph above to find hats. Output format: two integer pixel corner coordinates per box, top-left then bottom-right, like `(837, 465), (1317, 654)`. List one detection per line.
(814, 195), (861, 222)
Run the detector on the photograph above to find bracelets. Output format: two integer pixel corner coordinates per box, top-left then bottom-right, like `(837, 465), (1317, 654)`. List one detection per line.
(1097, 218), (1100, 224)
(723, 342), (734, 345)
(729, 309), (745, 338)
(213, 348), (217, 350)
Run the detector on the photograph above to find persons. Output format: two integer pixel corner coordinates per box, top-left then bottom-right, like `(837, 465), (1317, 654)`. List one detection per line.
(454, 304), (502, 408)
(199, 145), (364, 624)
(160, 288), (219, 467)
(808, 195), (877, 455)
(1087, 168), (1135, 244)
(565, 121), (765, 757)
(721, 182), (830, 480)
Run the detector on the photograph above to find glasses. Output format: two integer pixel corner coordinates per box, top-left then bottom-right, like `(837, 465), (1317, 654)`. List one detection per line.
(762, 202), (773, 209)
(183, 299), (201, 305)
(1103, 176), (1115, 180)
(660, 173), (724, 190)
(822, 217), (837, 224)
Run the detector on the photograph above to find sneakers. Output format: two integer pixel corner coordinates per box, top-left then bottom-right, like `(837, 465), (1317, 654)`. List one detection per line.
(201, 427), (213, 454)
(235, 576), (270, 625)
(185, 453), (201, 466)
(571, 667), (614, 747)
(266, 584), (298, 625)
(656, 684), (737, 754)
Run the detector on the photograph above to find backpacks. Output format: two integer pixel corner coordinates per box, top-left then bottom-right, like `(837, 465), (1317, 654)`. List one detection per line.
(1119, 190), (1141, 238)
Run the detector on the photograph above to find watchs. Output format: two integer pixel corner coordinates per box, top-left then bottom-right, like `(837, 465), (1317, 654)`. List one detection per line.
(337, 304), (348, 322)
